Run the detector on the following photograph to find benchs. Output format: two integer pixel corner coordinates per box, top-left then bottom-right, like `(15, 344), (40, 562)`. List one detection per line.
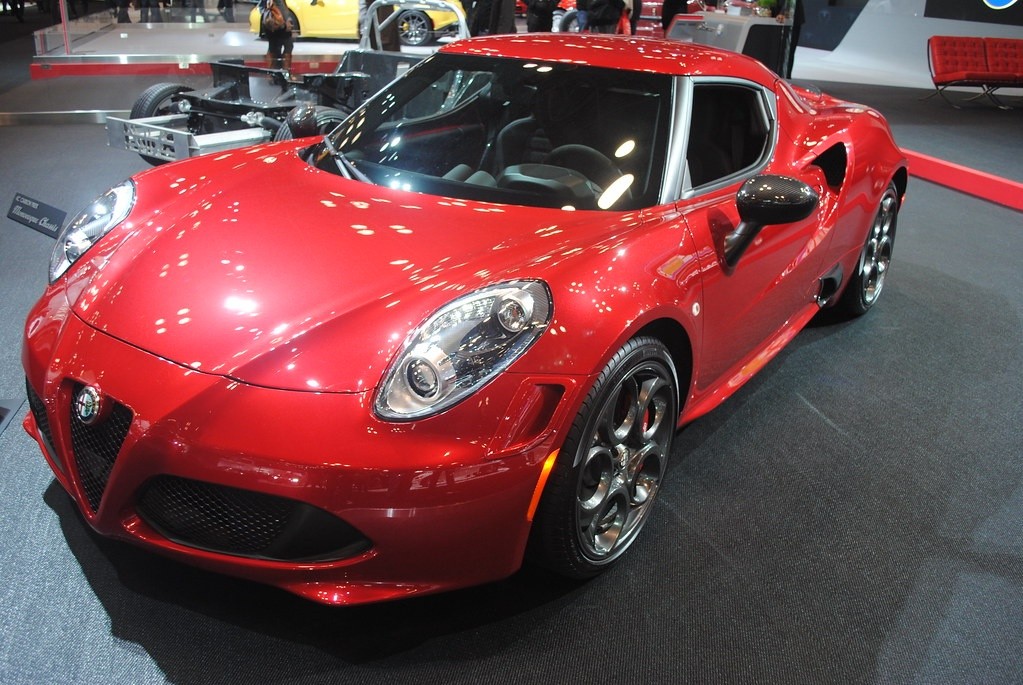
(919, 35), (1023, 112)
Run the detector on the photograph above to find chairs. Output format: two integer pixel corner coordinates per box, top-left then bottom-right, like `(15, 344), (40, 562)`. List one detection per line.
(610, 92), (733, 184)
(492, 79), (602, 174)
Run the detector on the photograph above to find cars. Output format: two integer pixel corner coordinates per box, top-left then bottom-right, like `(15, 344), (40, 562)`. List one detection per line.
(555, 0), (716, 38)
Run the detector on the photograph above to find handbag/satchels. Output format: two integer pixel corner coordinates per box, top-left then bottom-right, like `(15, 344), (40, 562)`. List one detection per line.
(264, 0), (285, 32)
(614, 10), (631, 36)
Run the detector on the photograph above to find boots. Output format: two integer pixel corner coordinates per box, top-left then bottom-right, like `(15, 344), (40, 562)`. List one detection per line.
(282, 53), (297, 80)
(266, 51), (279, 80)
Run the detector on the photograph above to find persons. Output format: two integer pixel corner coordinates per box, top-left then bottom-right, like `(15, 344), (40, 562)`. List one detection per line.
(356, 0), (805, 82)
(258, 0), (297, 80)
(0, 0), (234, 27)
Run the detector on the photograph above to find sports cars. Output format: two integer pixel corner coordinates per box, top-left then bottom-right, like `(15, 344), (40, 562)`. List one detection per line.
(248, 0), (468, 47)
(26, 33), (909, 619)
